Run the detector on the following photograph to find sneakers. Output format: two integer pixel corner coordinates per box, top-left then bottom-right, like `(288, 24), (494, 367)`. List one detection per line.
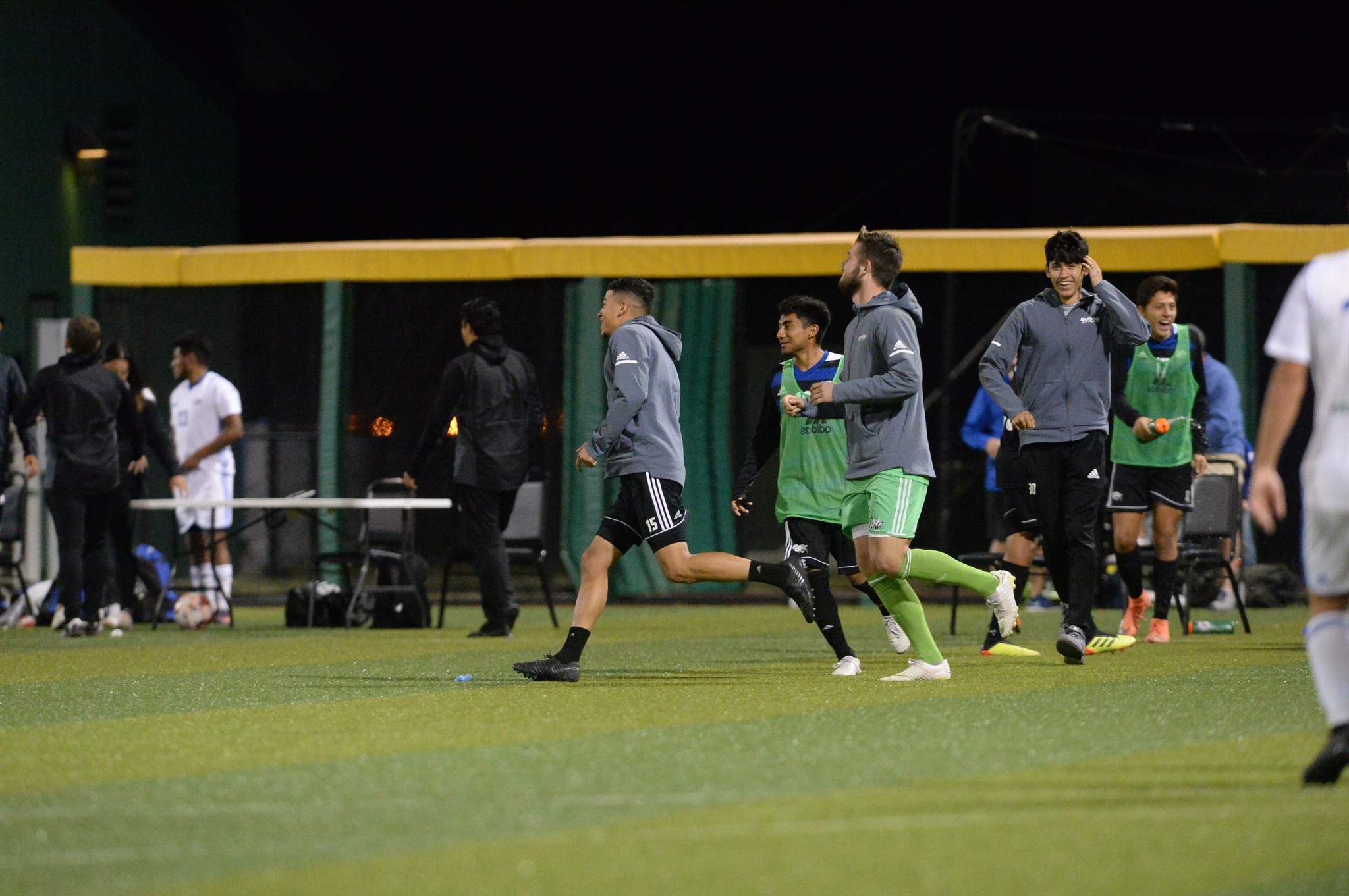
(1169, 594), (1186, 609)
(980, 638), (1041, 658)
(212, 610), (231, 625)
(831, 655), (862, 676)
(780, 554), (817, 624)
(1302, 723), (1349, 784)
(1085, 629), (1136, 656)
(985, 570), (1019, 639)
(880, 658), (952, 682)
(512, 657), (580, 683)
(1209, 579), (1247, 610)
(882, 614), (911, 655)
(50, 602), (133, 638)
(1012, 617), (1021, 633)
(1145, 588), (1156, 602)
(1118, 587), (1151, 637)
(1144, 618), (1169, 644)
(466, 597), (519, 637)
(1025, 593), (1058, 612)
(1056, 625), (1086, 665)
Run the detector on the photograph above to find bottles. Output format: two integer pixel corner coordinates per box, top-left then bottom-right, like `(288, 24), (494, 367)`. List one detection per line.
(1187, 620), (1235, 635)
(1135, 418), (1170, 444)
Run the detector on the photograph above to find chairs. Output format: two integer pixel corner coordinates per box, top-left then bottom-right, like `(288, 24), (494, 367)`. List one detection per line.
(308, 476), (427, 627)
(1134, 456), (1254, 638)
(433, 470), (563, 630)
(946, 550), (1047, 636)
(0, 469), (35, 617)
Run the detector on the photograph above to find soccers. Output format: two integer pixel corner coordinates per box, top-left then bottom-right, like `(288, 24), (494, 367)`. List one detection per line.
(175, 594), (212, 629)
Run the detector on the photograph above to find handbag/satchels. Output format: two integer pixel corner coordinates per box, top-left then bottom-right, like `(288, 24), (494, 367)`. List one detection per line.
(285, 581), (352, 627)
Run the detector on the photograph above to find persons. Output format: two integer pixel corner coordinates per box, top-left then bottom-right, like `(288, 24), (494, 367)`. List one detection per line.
(0, 316), (45, 632)
(11, 312), (150, 638)
(403, 295), (560, 639)
(1247, 250), (1349, 791)
(98, 339), (168, 633)
(1096, 319), (1256, 611)
(728, 296), (911, 676)
(979, 229), (1151, 665)
(164, 332), (250, 627)
(1098, 273), (1211, 647)
(780, 223), (1022, 683)
(961, 309), (1136, 661)
(511, 276), (820, 684)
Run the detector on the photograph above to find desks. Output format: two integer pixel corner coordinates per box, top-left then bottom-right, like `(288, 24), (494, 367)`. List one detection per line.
(126, 497), (457, 627)
